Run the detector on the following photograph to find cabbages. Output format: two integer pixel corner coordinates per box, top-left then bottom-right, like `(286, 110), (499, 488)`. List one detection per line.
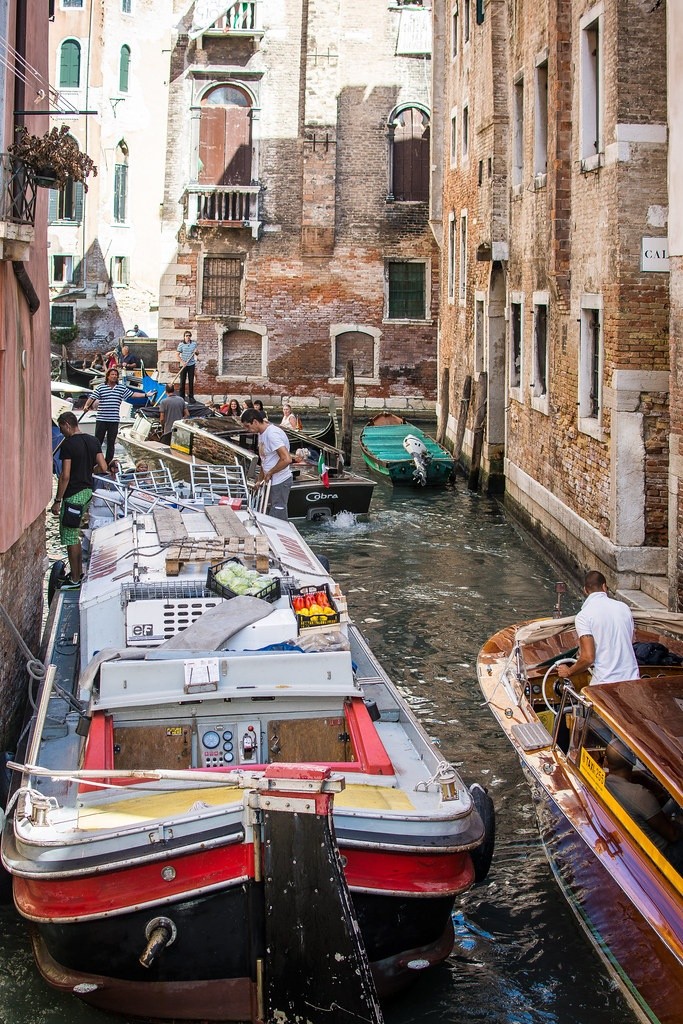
(214, 562), (277, 598)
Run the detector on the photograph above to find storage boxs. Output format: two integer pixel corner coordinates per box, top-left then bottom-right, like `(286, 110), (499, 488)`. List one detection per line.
(205, 557), (282, 607)
(288, 583), (341, 637)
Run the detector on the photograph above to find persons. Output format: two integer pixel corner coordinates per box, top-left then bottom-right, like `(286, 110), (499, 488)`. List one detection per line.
(295, 448), (311, 474)
(240, 408), (293, 521)
(51, 412), (107, 590)
(83, 369), (157, 464)
(210, 403), (227, 417)
(227, 399), (243, 416)
(280, 404), (297, 430)
(240, 400), (254, 417)
(604, 738), (683, 878)
(96, 459), (154, 491)
(159, 384), (189, 434)
(556, 571), (641, 684)
(90, 346), (136, 371)
(254, 400), (267, 421)
(126, 325), (148, 337)
(176, 331), (199, 403)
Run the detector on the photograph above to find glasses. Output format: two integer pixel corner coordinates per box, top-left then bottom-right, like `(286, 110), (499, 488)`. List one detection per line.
(185, 334), (190, 337)
(115, 466), (122, 469)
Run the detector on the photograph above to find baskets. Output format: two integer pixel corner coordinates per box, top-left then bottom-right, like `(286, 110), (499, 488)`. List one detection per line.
(289, 583), (340, 628)
(206, 557), (281, 603)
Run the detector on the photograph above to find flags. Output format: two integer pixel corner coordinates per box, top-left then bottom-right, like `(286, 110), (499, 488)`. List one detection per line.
(298, 417), (303, 430)
(318, 453), (329, 488)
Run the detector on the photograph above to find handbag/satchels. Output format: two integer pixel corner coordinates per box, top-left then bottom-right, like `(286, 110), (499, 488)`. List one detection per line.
(62, 502), (82, 528)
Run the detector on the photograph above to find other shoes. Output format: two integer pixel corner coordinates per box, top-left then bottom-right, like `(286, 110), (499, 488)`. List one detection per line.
(189, 397), (196, 403)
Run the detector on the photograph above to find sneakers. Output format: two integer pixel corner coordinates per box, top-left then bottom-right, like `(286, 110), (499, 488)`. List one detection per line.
(58, 572), (84, 581)
(53, 579), (81, 591)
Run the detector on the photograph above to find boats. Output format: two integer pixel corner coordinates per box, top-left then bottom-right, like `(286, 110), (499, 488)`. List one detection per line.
(477, 581), (683, 1024)
(0, 460), (495, 1024)
(51, 335), (378, 519)
(359, 408), (456, 485)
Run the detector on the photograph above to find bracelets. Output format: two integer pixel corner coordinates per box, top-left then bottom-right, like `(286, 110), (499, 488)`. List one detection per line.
(126, 365), (127, 366)
(145, 393), (146, 397)
(54, 499), (61, 504)
